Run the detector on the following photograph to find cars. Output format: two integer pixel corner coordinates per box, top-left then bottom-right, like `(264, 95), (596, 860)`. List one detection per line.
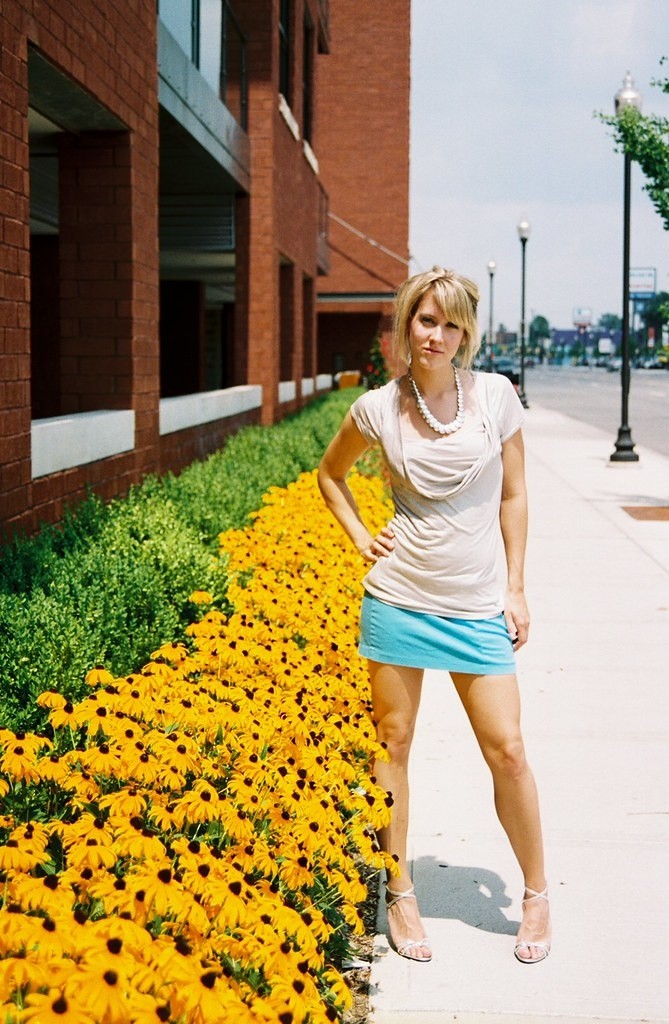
(492, 355), (520, 385)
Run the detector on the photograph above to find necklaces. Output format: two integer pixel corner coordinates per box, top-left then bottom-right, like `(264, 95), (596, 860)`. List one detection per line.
(405, 359), (467, 434)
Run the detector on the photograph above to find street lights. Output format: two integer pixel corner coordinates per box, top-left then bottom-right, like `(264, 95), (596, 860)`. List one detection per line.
(516, 216), (530, 410)
(609, 64), (641, 462)
(487, 262), (496, 372)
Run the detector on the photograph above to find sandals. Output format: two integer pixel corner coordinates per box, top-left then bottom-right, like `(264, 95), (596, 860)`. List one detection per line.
(385, 884), (432, 962)
(514, 885), (550, 963)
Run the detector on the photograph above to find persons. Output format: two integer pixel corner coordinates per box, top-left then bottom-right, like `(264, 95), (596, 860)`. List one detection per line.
(317, 268), (552, 965)
